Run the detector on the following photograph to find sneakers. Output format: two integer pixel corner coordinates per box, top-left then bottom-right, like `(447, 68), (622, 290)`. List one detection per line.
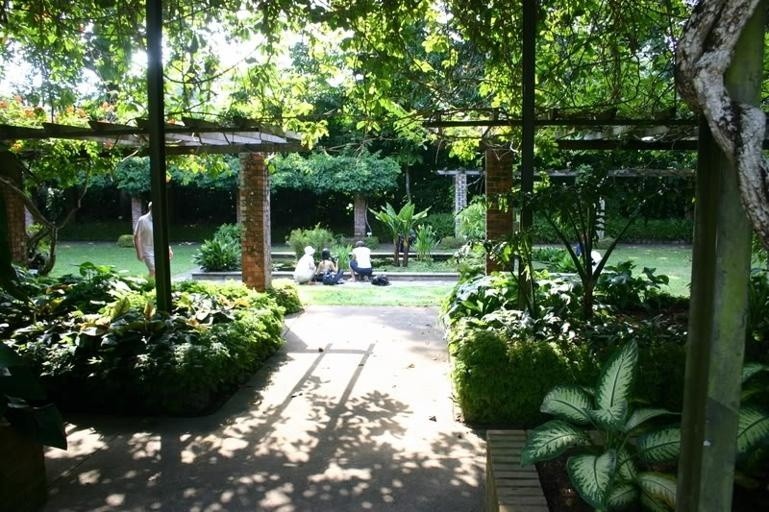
(348, 278), (356, 282)
(363, 275), (369, 282)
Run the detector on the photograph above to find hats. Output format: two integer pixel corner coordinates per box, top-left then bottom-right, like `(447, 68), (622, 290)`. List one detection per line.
(304, 246), (315, 255)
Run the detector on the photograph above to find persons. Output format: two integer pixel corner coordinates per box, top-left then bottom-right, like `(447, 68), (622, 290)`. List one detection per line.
(314, 248), (344, 284)
(398, 228), (417, 252)
(576, 233), (587, 257)
(346, 241), (372, 282)
(293, 245), (317, 285)
(134, 201), (174, 277)
